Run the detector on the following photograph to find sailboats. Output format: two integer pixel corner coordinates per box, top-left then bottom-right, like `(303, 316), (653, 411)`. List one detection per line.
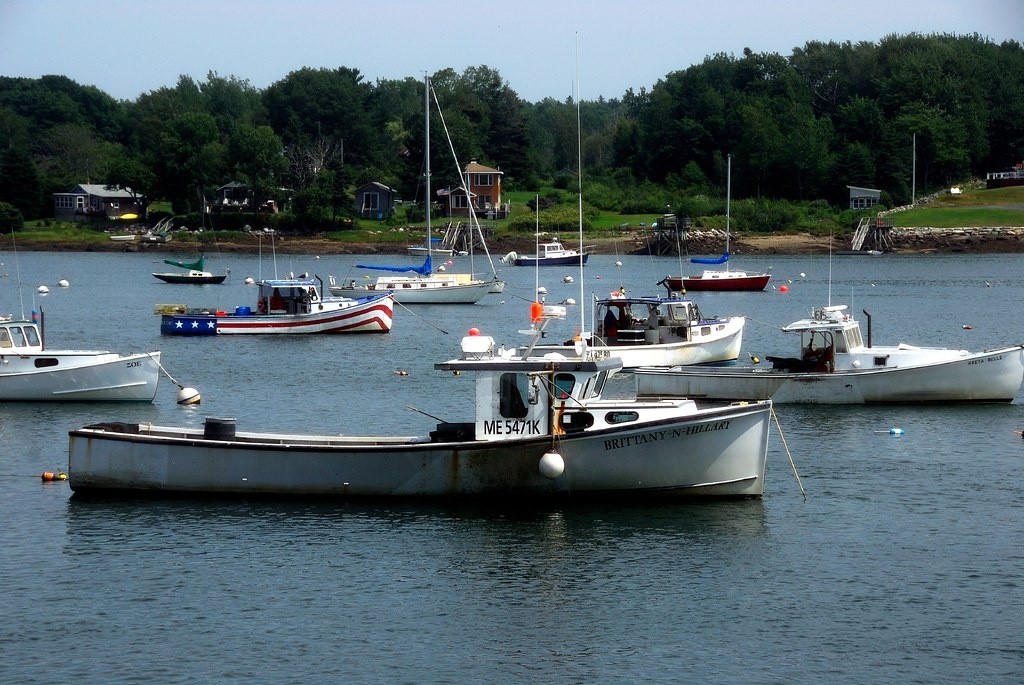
(324, 67), (501, 305)
(152, 193), (230, 285)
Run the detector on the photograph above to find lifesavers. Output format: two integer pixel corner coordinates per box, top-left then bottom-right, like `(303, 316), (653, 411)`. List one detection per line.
(216, 312), (227, 316)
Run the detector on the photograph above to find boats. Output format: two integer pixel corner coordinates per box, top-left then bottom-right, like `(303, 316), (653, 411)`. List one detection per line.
(64, 302), (777, 504)
(1, 227), (165, 404)
(515, 220), (746, 370)
(153, 227), (396, 337)
(110, 234), (136, 241)
(656, 151), (773, 292)
(406, 236), (454, 257)
(498, 221), (597, 267)
(634, 227), (1024, 405)
